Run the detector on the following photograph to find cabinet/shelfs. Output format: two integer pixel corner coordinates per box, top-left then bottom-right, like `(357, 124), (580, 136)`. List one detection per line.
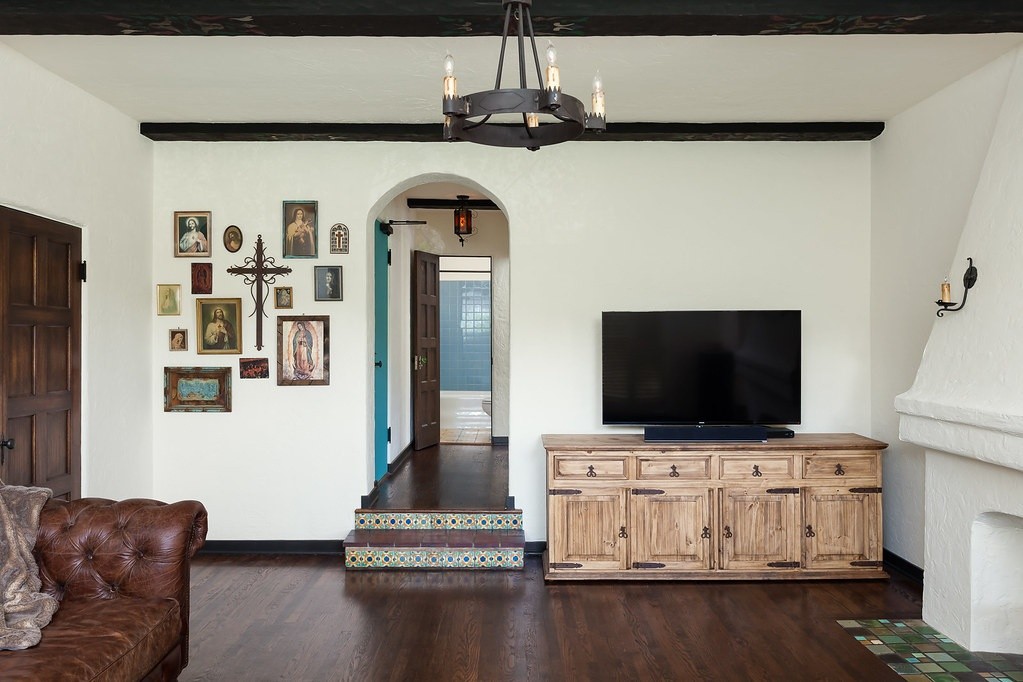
(542, 433), (891, 581)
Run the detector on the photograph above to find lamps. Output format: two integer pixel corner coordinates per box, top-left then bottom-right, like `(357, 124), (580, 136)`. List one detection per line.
(443, 0), (607, 152)
(454, 194), (479, 247)
(934, 257), (977, 318)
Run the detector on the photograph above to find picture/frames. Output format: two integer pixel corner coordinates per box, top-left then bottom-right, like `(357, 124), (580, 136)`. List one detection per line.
(169, 329), (188, 352)
(196, 298), (242, 355)
(157, 283), (181, 316)
(277, 315), (330, 386)
(282, 200), (318, 259)
(314, 266), (343, 301)
(174, 211), (212, 258)
(274, 287), (293, 309)
(164, 367), (232, 413)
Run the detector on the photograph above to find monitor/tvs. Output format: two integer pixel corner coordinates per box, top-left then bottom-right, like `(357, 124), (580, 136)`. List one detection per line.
(601, 310), (801, 428)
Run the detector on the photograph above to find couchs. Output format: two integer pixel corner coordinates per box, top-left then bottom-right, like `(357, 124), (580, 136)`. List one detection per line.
(0, 483), (208, 682)
